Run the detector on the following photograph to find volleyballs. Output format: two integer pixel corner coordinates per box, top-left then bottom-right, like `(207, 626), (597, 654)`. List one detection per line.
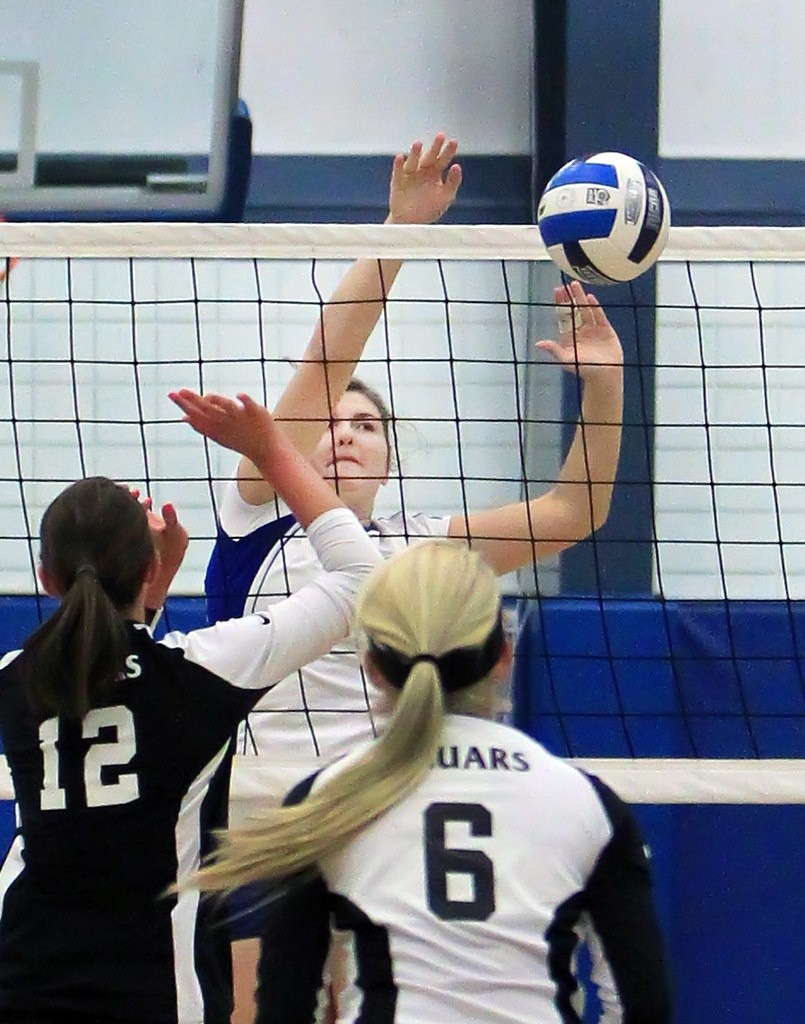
(534, 151), (673, 284)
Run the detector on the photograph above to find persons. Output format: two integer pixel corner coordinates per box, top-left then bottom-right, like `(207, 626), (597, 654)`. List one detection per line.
(0, 384), (389, 1023)
(201, 125), (626, 1022)
(162, 532), (673, 1023)
